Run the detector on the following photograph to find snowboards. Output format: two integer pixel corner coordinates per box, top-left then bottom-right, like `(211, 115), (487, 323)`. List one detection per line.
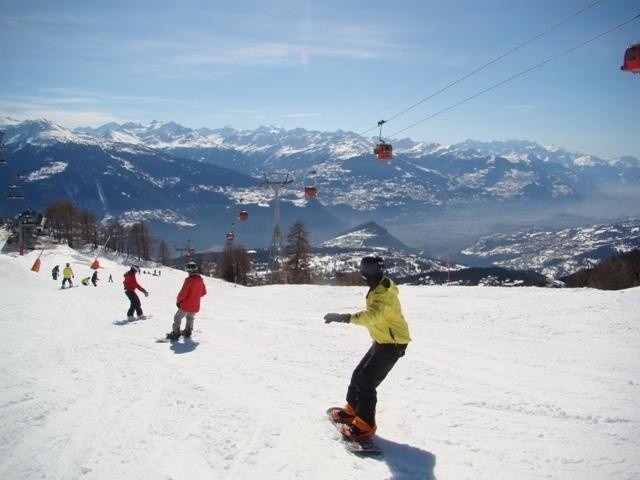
(156, 329), (202, 343)
(327, 407), (381, 453)
(60, 285), (79, 289)
(113, 313), (154, 325)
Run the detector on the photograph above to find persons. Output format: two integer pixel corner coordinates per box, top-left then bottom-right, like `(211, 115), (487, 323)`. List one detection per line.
(51, 263), (161, 321)
(323, 256), (412, 438)
(166, 261), (207, 340)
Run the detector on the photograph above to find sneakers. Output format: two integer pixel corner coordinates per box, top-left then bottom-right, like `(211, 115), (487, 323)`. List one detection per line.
(340, 416), (376, 441)
(180, 330), (191, 337)
(328, 404), (357, 425)
(167, 333), (179, 340)
(127, 315), (135, 320)
(138, 315), (146, 318)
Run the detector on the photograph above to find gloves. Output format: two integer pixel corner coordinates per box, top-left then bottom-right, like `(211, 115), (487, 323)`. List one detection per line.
(324, 314), (351, 324)
(144, 290), (148, 297)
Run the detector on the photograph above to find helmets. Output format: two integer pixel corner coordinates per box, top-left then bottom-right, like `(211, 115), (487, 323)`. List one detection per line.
(360, 257), (383, 286)
(131, 265), (139, 272)
(185, 262), (197, 271)
